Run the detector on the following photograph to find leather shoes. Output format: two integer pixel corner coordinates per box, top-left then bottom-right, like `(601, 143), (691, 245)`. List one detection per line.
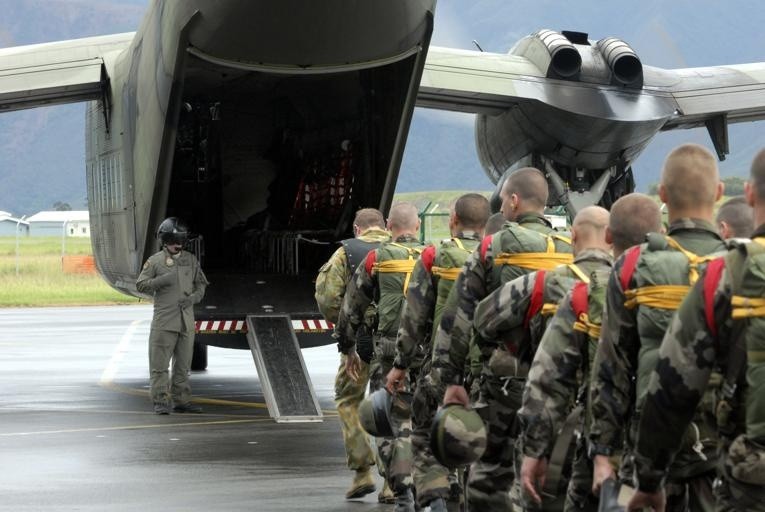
(173, 401), (204, 414)
(153, 401), (169, 414)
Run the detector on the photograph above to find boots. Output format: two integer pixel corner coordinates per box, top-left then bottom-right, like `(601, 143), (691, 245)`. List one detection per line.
(344, 468), (376, 498)
(430, 497), (449, 512)
(392, 487), (425, 512)
(377, 476), (397, 504)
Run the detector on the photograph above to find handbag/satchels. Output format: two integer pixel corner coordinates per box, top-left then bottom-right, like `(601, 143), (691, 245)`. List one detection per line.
(482, 349), (531, 409)
(716, 435), (764, 509)
(373, 332), (398, 360)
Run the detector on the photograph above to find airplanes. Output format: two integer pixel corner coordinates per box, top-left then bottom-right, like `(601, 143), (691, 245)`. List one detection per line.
(0, 0), (765, 433)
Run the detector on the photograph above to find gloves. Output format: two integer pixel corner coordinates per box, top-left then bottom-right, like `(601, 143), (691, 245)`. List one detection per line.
(153, 273), (176, 290)
(178, 290), (201, 309)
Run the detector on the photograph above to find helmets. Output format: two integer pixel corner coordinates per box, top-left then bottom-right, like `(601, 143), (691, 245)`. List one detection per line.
(429, 399), (488, 469)
(156, 217), (189, 242)
(358, 386), (402, 439)
(585, 476), (656, 511)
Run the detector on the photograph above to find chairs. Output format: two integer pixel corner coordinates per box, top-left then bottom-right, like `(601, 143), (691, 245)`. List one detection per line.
(247, 202), (351, 279)
(183, 229), (205, 273)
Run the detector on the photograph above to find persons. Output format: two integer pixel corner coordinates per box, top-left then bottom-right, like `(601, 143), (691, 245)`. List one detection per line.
(136, 216), (209, 415)
(314, 143), (765, 512)
